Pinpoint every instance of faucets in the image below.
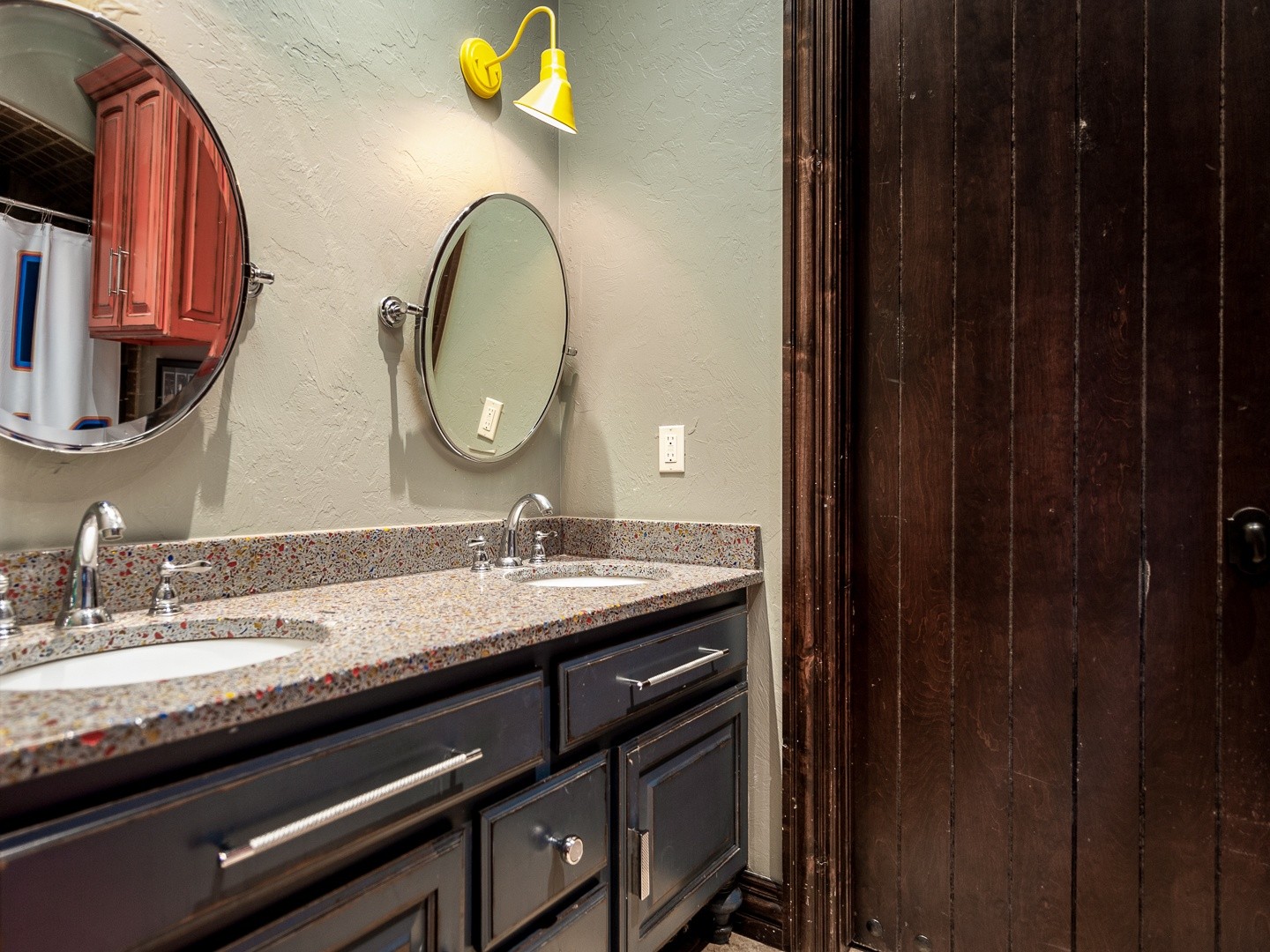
[50,501,126,631]
[489,492,554,569]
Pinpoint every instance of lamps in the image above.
[460,6,577,134]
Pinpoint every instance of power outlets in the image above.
[658,425,686,473]
[477,396,504,442]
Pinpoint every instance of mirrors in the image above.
[0,0,274,457]
[378,192,578,466]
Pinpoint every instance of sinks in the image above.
[0,613,333,693]
[505,559,675,588]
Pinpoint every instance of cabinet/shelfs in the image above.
[71,53,245,346]
[0,585,752,951]
